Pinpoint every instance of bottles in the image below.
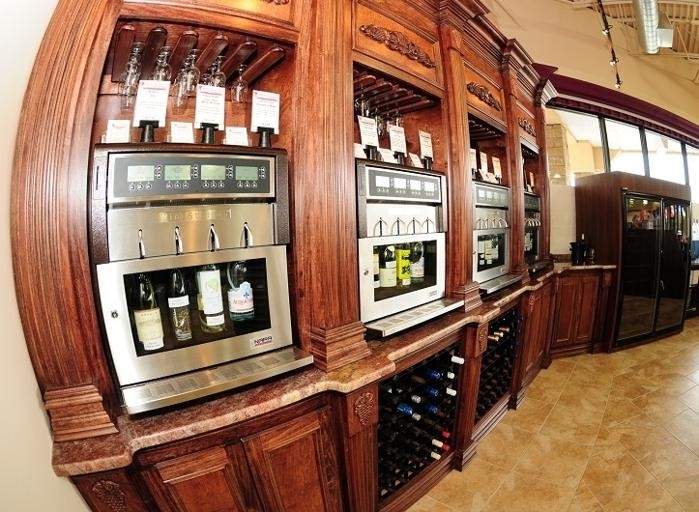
[226,261,257,323]
[127,272,166,352]
[372,241,426,291]
[193,263,227,335]
[396,326,512,464]
[166,267,194,344]
[479,234,500,266]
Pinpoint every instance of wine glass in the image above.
[115,42,250,111]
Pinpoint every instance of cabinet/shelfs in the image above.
[521,271,607,383]
[573,170,693,355]
[122,402,351,510]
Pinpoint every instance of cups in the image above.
[354,84,405,138]
